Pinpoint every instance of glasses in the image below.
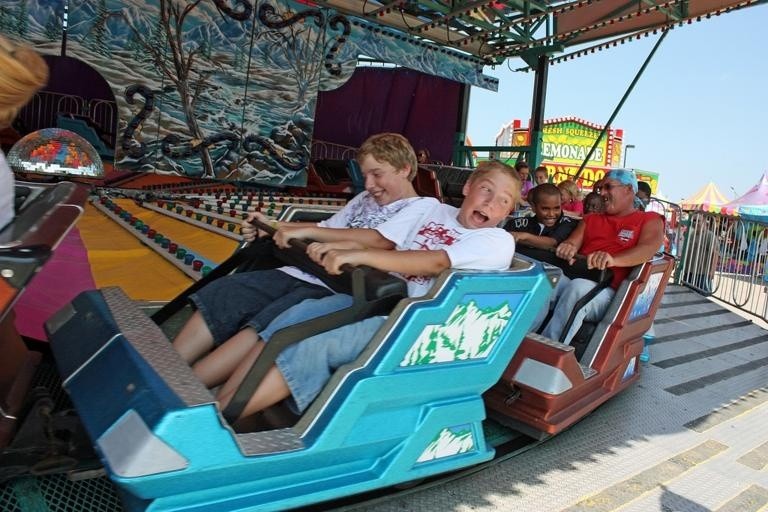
[595,184,627,192]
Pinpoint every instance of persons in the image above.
[213,159,525,420]
[0,35,50,234]
[170,131,437,389]
[497,160,668,343]
[664,211,768,292]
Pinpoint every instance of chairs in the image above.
[220,213,674,450]
